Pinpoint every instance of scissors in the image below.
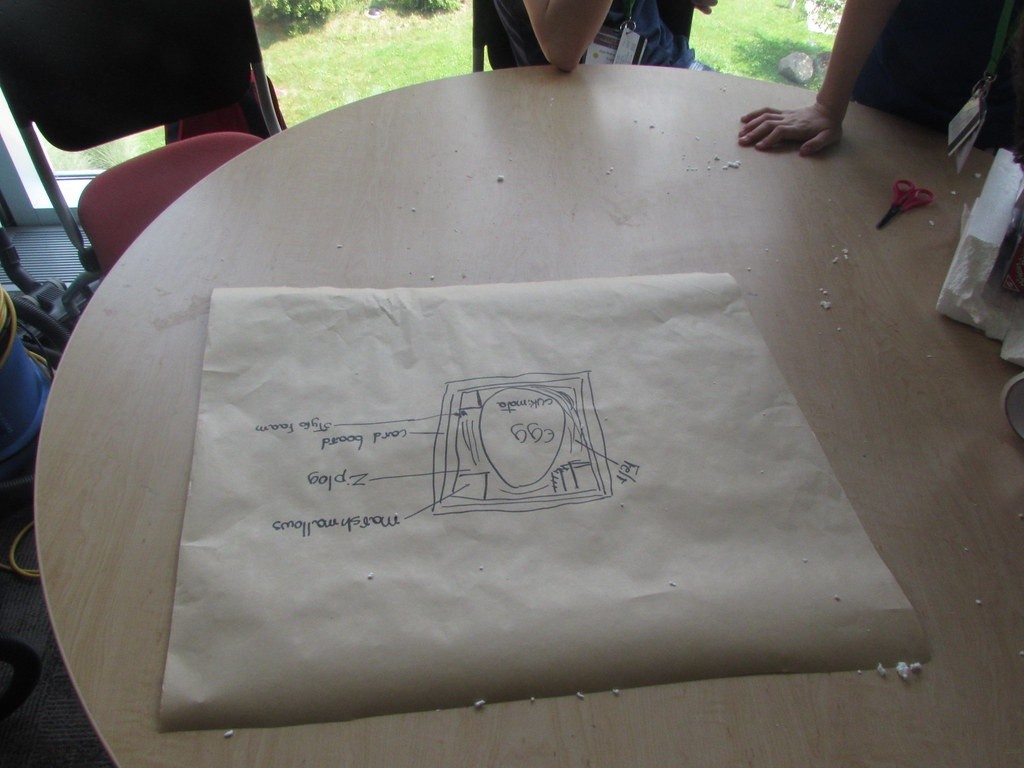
[876,179,936,230]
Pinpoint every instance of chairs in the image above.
[1,0,288,322]
[473,0,694,74]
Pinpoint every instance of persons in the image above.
[737,1,1024,156]
[486,0,720,74]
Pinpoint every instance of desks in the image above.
[32,63,1022,768]
